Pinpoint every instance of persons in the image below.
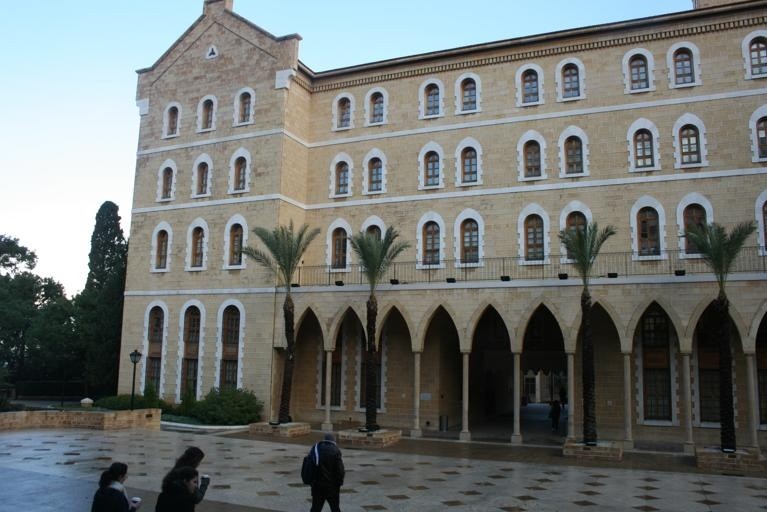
[307,432,344,512]
[559,386,568,410]
[548,400,561,434]
[155,465,198,512]
[162,447,210,504]
[91,462,142,512]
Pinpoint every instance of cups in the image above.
[131,497,141,510]
[201,475,211,486]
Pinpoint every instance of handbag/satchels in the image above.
[301,456,312,485]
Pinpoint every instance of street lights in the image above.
[129,349,142,410]
